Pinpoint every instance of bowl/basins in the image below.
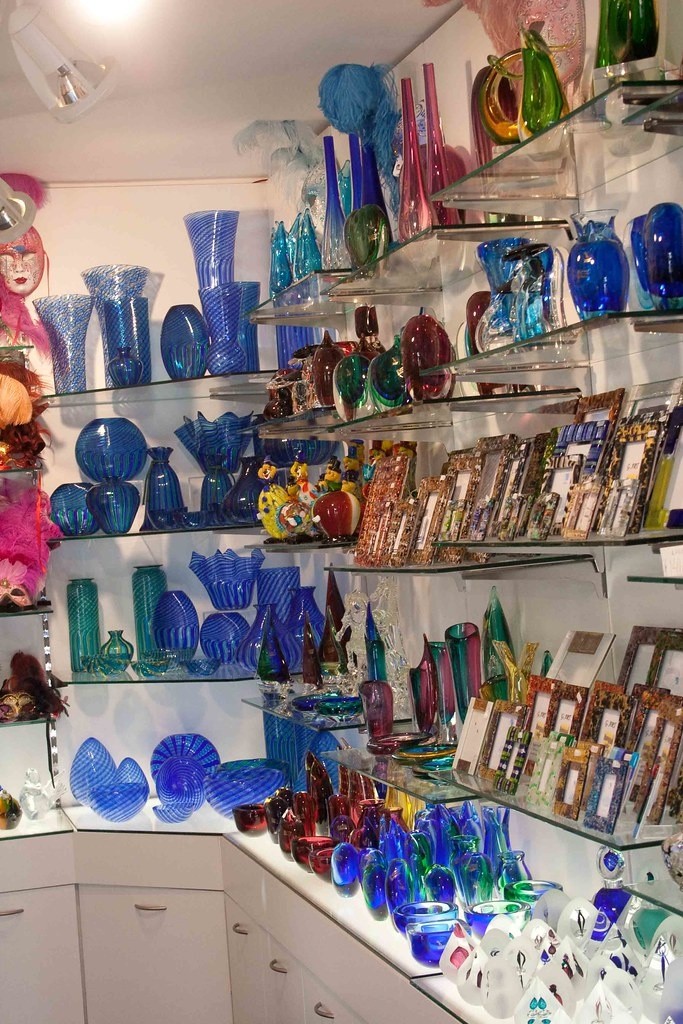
[81,649,223,680]
[308,847,361,884]
[391,901,533,965]
[293,836,333,871]
[233,803,267,837]
[147,504,211,530]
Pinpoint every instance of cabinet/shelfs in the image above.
[34,368,278,833]
[224,891,266,1024]
[240,269,418,734]
[303,961,368,1024]
[77,882,233,1024]
[619,84,683,916]
[309,220,615,806]
[263,928,306,1024]
[0,883,85,1024]
[0,344,74,844]
[429,79,683,853]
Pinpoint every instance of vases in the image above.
[278,745,565,968]
[65,547,362,725]
[33,210,262,395]
[269,62,463,296]
[358,583,553,747]
[48,411,338,537]
[69,690,333,884]
[265,202,683,423]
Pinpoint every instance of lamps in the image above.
[0,177,36,243]
[7,0,121,124]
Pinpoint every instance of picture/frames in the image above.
[451,626,683,836]
[354,376,683,568]
